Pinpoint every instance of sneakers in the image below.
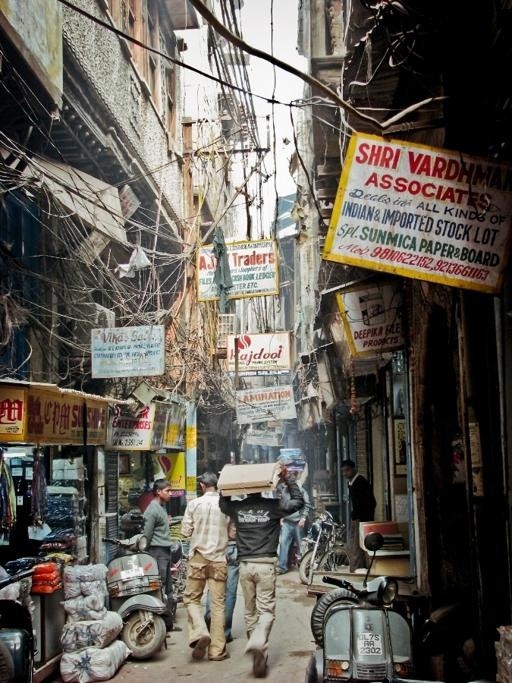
[166,627,268,678]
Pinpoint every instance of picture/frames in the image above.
[393,416,407,477]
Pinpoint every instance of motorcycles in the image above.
[304,532,432,682]
[96,534,188,659]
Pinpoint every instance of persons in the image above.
[339,460,377,573]
[218,460,305,678]
[203,521,238,642]
[180,471,235,661]
[142,478,181,631]
[275,466,310,575]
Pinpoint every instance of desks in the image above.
[362,544,410,576]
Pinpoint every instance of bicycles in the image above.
[298,504,353,585]
